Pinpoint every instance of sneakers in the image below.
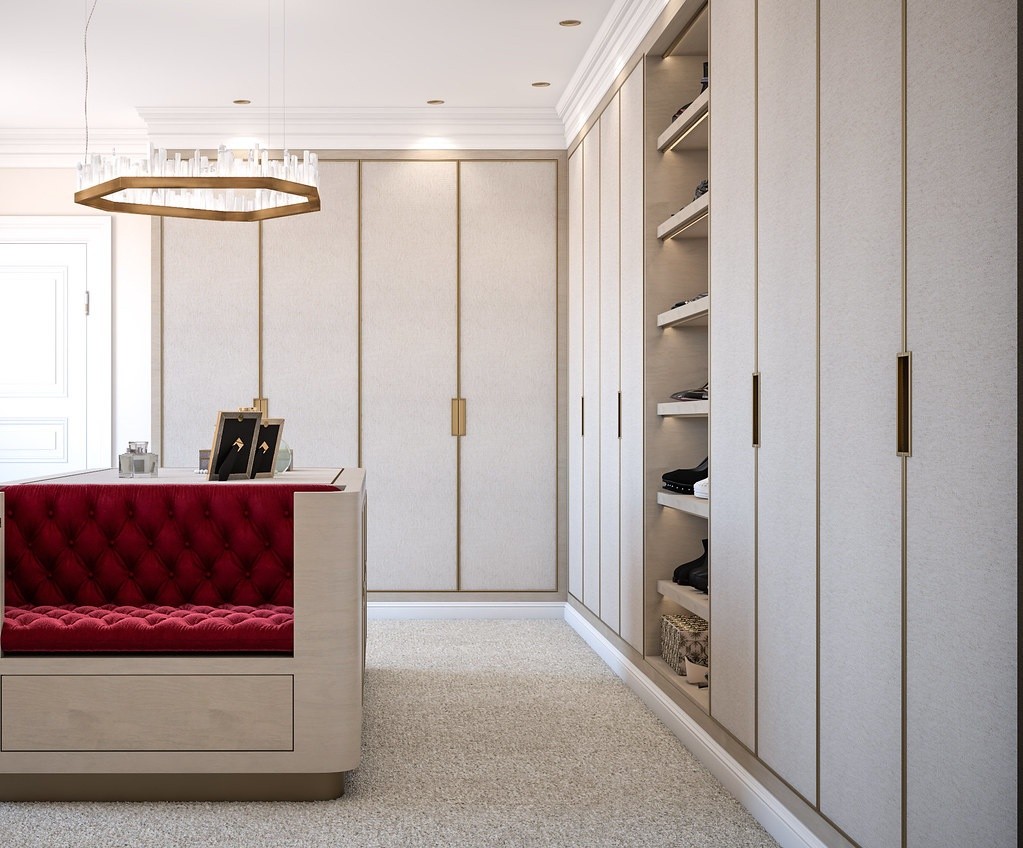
[670,380,709,401]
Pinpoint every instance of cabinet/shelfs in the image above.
[150,1,1023,847]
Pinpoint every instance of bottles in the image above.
[118,441,159,478]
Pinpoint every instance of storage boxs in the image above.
[662,614,709,675]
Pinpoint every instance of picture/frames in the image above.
[208,411,262,481]
[250,418,285,478]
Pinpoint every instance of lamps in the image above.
[74,1,321,222]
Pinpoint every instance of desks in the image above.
[0,467,366,804]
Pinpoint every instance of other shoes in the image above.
[661,456,709,495]
[671,539,708,593]
[693,477,709,500]
